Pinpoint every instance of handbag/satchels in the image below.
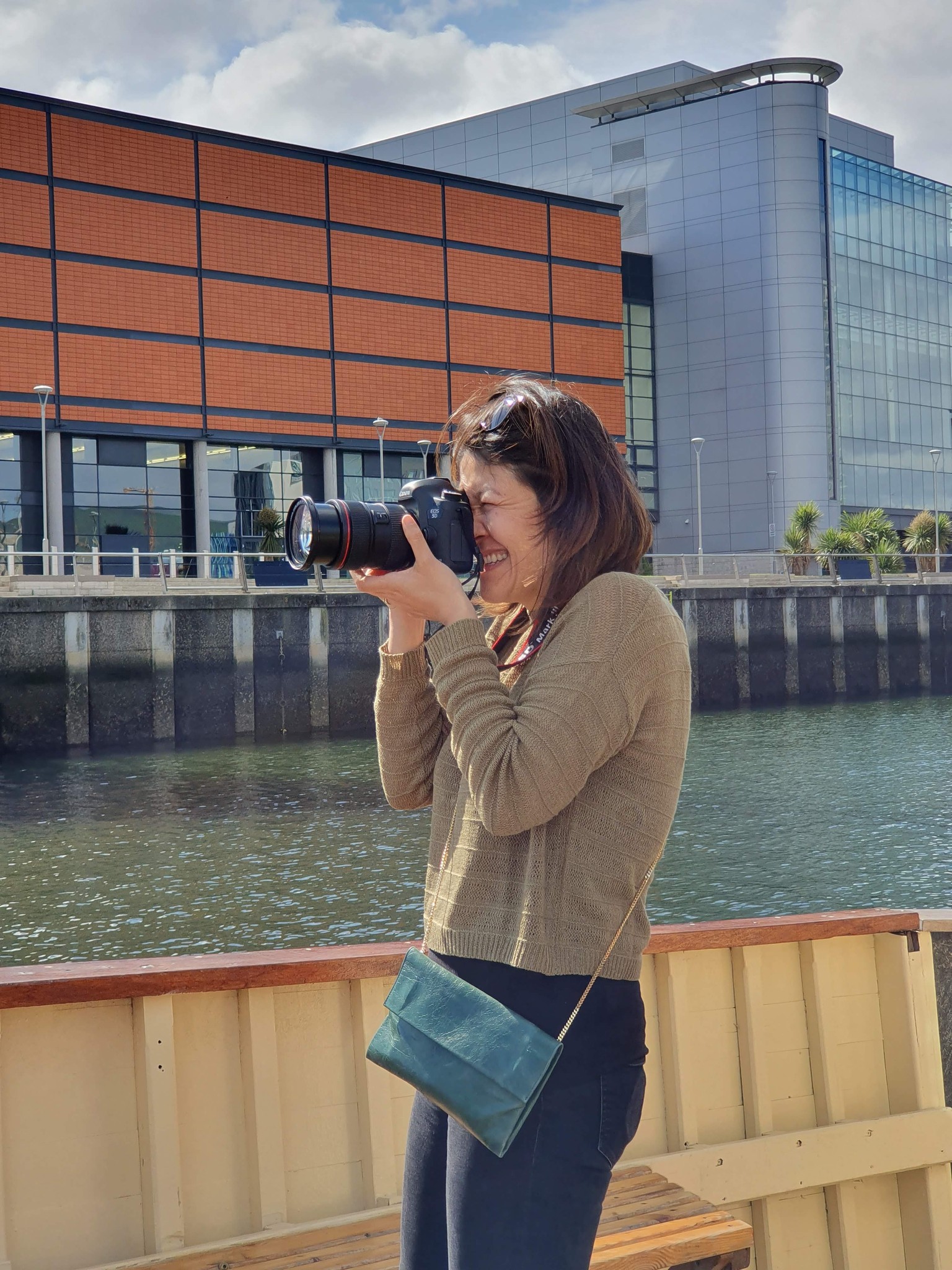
[366,947,563,1158]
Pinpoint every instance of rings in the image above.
[383,598,390,606]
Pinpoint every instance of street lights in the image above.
[418,440,434,479]
[372,416,389,504]
[766,470,778,554]
[34,385,54,576]
[929,448,942,573]
[691,437,705,575]
[0,500,9,552]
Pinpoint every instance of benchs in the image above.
[89,1165,756,1270]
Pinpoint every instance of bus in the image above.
[150,557,184,577]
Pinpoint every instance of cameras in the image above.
[284,476,474,576]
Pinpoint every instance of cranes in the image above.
[124,488,155,552]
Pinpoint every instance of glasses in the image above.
[474,392,532,431]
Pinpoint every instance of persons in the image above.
[347,377,694,1270]
